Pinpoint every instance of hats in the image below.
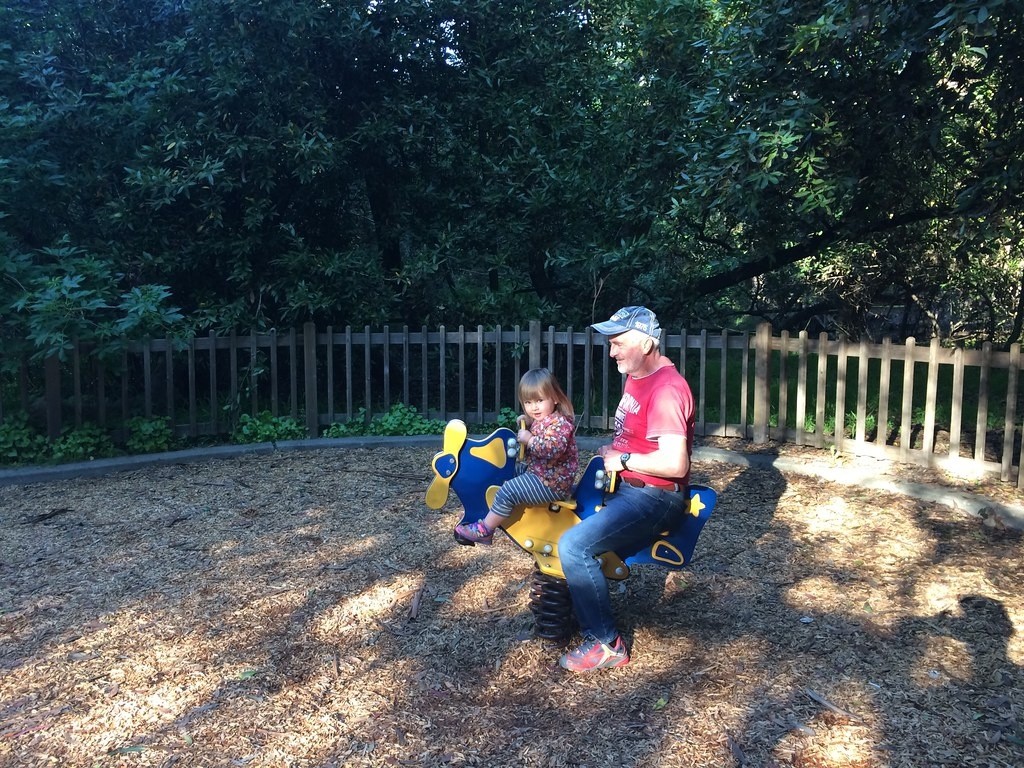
[591,306,662,340]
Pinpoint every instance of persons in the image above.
[455,368,579,545]
[557,306,695,671]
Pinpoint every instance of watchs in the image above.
[620,452,631,471]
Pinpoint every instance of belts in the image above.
[618,475,684,491]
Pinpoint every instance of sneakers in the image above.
[456,519,493,545]
[559,636,628,672]
